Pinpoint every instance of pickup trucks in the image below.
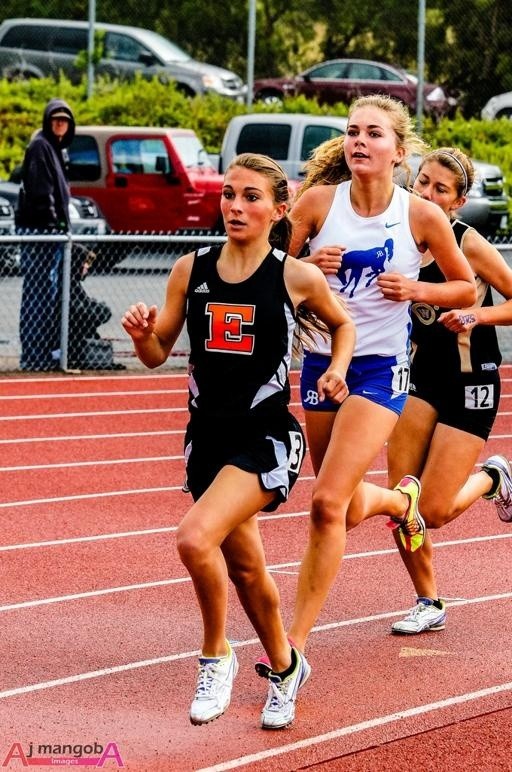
[137,110,505,243]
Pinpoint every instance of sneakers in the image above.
[391,597,446,635]
[254,641,311,728]
[189,640,239,726]
[386,474,425,554]
[482,455,512,524]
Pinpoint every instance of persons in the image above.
[254,92,477,682]
[15,100,75,372]
[122,152,357,728]
[391,149,512,634]
[67,243,112,369]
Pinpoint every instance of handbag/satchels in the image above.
[80,339,114,370]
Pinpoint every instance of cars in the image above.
[481,90,512,125]
[0,182,120,278]
[255,56,461,123]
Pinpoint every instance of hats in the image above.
[51,109,72,121]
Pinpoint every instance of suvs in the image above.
[8,121,304,265]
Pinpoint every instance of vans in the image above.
[0,18,251,104]
